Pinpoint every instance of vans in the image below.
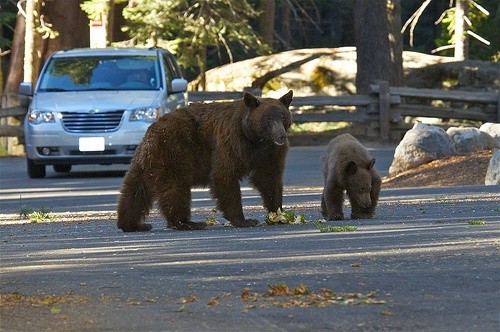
[19,46,190,179]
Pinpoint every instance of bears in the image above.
[320,133,382,221]
[115,90,294,233]
